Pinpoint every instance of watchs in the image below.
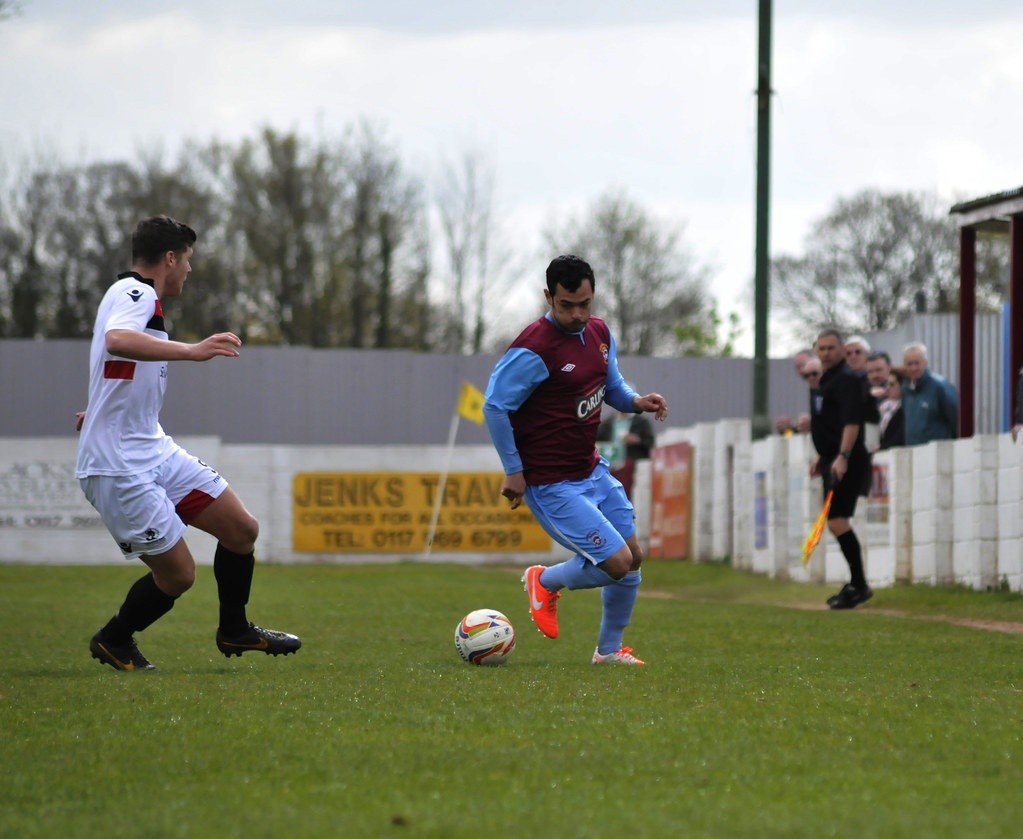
[840,452,850,458]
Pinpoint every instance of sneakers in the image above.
[591,646,646,665]
[90,629,156,672]
[826,583,872,609]
[216,622,302,658]
[521,565,561,639]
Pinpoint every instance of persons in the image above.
[878,369,905,450]
[596,411,654,501]
[482,255,669,665]
[807,329,873,609]
[775,351,823,437]
[900,343,960,447]
[73,214,302,670]
[845,335,892,403]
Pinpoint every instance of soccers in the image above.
[454,608,516,666]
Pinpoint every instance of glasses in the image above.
[846,350,860,356]
[803,371,818,379]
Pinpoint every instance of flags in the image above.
[458,384,486,426]
[803,504,830,566]
